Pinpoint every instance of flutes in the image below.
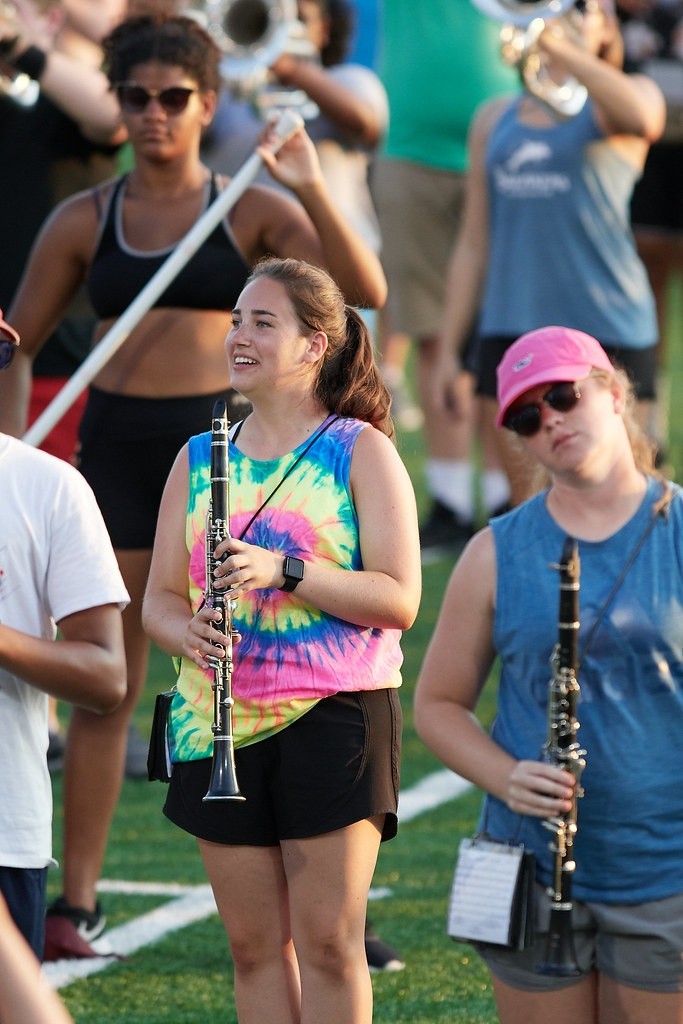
[190,397,247,806]
[527,538,588,981]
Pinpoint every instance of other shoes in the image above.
[414,497,517,561]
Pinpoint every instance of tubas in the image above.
[475,0,604,117]
[203,0,325,127]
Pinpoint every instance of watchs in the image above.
[277,555,304,593]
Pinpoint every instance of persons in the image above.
[411,326,682,1023]
[431,0,666,511]
[0,16,408,971]
[368,0,527,558]
[204,0,388,257]
[0,305,132,974]
[141,258,421,1024]
[0,1,120,758]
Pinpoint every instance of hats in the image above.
[496,327,614,430]
[0,307,20,347]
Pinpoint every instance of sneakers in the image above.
[47,896,106,945]
[364,940,406,973]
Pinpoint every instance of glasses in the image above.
[116,84,203,116]
[0,340,16,371]
[575,0,594,17]
[506,371,607,435]
